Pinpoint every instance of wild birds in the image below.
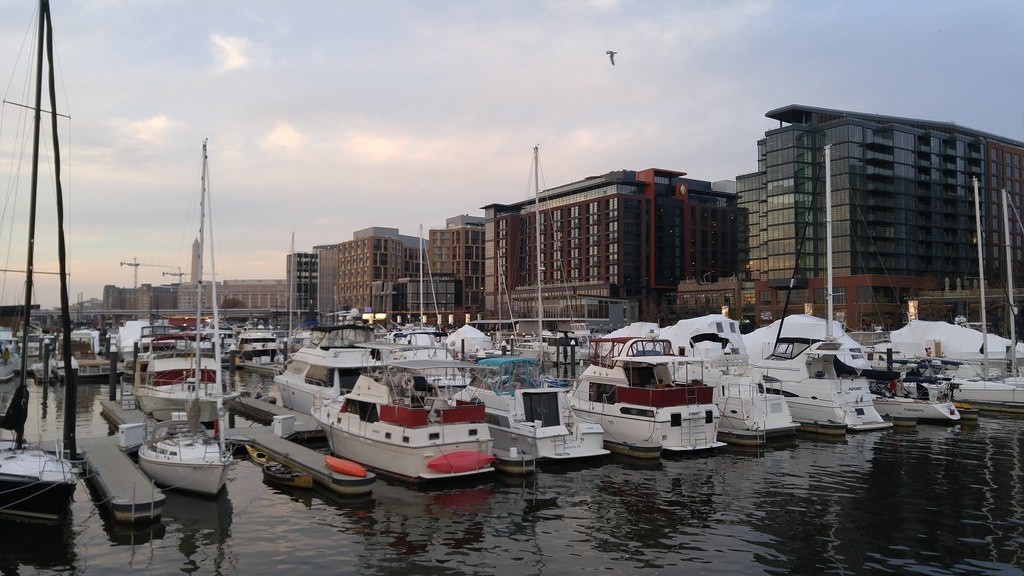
[605,50,618,66]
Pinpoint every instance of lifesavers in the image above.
[400,374,415,388]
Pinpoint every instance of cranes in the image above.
[163,267,219,283]
[120,258,173,286]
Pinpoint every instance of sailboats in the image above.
[138,137,239,494]
[0,0,89,527]
[514,146,601,362]
[383,223,448,343]
[750,144,961,419]
[896,175,1024,402]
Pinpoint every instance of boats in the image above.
[135,333,242,423]
[447,357,611,459]
[383,330,473,387]
[752,342,893,430]
[668,355,801,436]
[13,333,41,373]
[566,356,728,450]
[311,359,498,481]
[0,339,21,381]
[244,445,279,465]
[230,330,278,364]
[273,324,387,415]
[53,331,125,380]
[263,462,313,489]
